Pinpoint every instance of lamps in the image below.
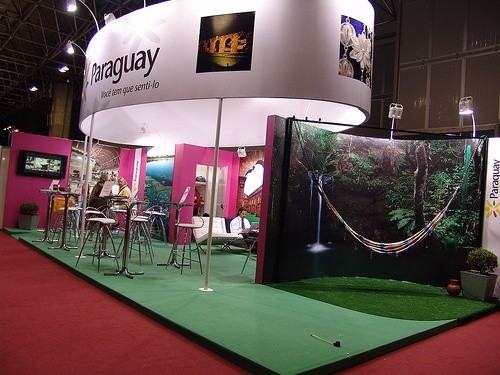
[388,103,404,138]
[58,65,69,73]
[459,97,475,137]
[237,146,247,158]
[67,40,85,58]
[66,0,100,33]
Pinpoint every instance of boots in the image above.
[88,232,94,241]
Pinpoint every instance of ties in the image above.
[241,218,245,232]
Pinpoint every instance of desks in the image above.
[157,202,196,269]
[33,188,80,252]
[101,200,149,279]
[238,230,259,273]
[82,195,128,260]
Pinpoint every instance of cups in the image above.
[129,198,137,218]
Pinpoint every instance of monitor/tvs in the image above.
[16,150,68,179]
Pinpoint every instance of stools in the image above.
[165,216,204,274]
[47,206,168,273]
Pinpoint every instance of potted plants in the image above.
[18,203,40,229]
[460,248,498,300]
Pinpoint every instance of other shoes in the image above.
[109,222,120,231]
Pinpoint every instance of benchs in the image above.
[192,216,249,254]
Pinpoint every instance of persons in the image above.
[194,189,201,215]
[88,174,112,241]
[113,177,131,233]
[230,207,258,253]
